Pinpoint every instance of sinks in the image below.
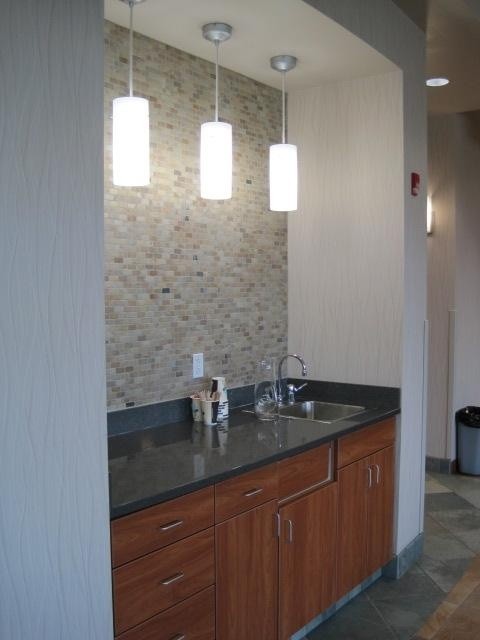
[242,398,379,425]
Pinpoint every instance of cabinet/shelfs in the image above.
[339,418,396,606]
[212,439,340,640]
[112,482,215,639]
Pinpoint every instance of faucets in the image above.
[275,353,306,405]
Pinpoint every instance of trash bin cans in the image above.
[456,406,480,476]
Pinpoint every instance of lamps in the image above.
[108,0,153,190]
[196,21,234,203]
[268,51,301,212]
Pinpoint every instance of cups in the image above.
[255,359,280,424]
[190,377,229,425]
